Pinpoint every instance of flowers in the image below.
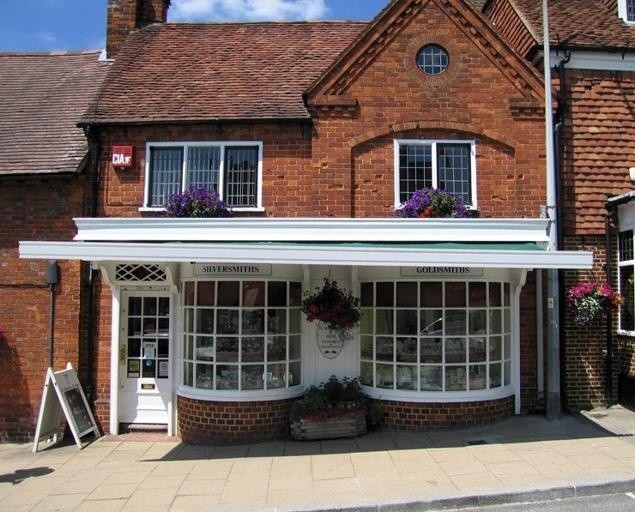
[292,372,371,422]
[160,184,235,218]
[566,280,626,327]
[394,185,470,218]
[300,276,362,333]
